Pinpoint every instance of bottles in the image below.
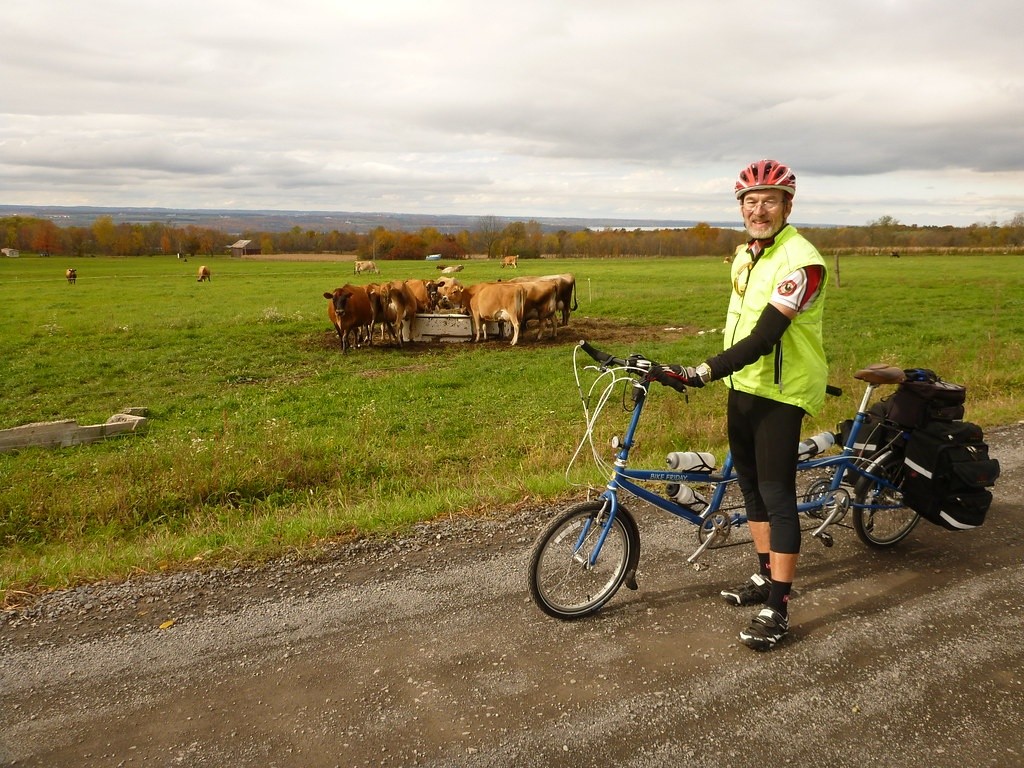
[798,431,836,460]
[666,483,705,511]
[666,452,715,471]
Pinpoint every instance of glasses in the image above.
[741,199,785,211]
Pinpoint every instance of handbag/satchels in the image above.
[840,368,1000,532]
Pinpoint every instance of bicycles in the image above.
[528,340,922,621]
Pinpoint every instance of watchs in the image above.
[696,365,712,383]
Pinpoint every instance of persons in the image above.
[645,160,829,651]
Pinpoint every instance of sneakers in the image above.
[738,605,789,648]
[721,573,772,607]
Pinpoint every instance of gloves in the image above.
[644,364,706,388]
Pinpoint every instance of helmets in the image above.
[735,159,797,200]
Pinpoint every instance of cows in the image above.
[497,274,578,341]
[66,268,77,284]
[436,264,464,273]
[500,255,519,269]
[354,261,380,275]
[323,276,459,355]
[443,282,526,345]
[197,266,211,281]
